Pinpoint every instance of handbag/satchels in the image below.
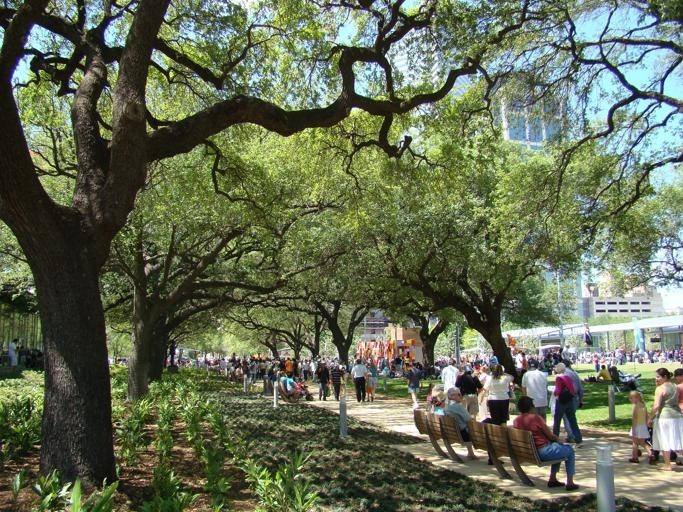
[558,385,575,405]
[508,385,516,399]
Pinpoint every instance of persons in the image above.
[382,347,585,491]
[586,347,683,470]
[8,338,43,369]
[171,347,377,403]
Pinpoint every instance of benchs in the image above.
[468,419,568,486]
[264,379,299,403]
[412,408,472,464]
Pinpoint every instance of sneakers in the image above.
[576,441,584,448]
[412,404,419,410]
[630,458,639,462]
[548,480,565,487]
[650,455,656,465]
[566,484,579,490]
[487,458,504,465]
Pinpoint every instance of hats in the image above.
[527,358,539,368]
